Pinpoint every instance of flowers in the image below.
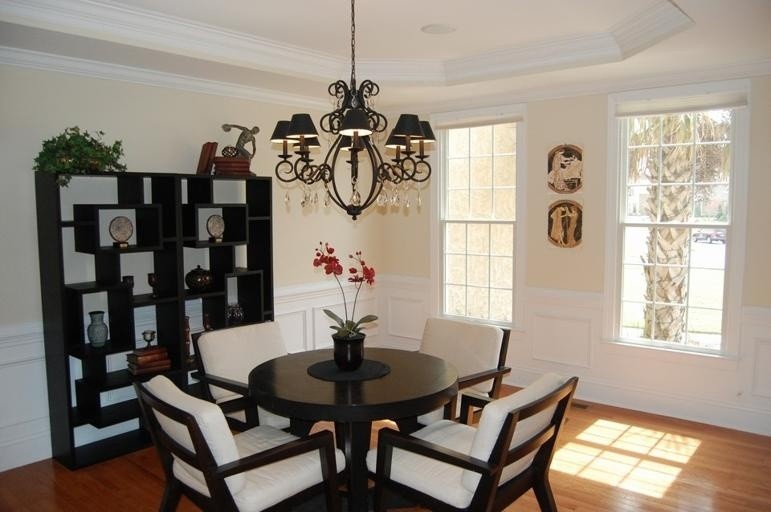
[312,241,378,337]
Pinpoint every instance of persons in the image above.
[551,148,577,190]
[222,123,260,160]
[551,203,578,245]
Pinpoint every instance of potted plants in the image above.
[33,126,127,185]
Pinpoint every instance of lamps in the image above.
[270,0,437,221]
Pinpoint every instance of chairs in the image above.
[363,371,579,512]
[134,374,346,512]
[413,317,511,428]
[191,321,291,433]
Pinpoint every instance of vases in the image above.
[331,332,366,371]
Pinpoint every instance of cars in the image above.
[693,228,727,245]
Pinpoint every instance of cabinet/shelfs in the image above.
[35,171,274,469]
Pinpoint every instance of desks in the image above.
[249,346,458,512]
